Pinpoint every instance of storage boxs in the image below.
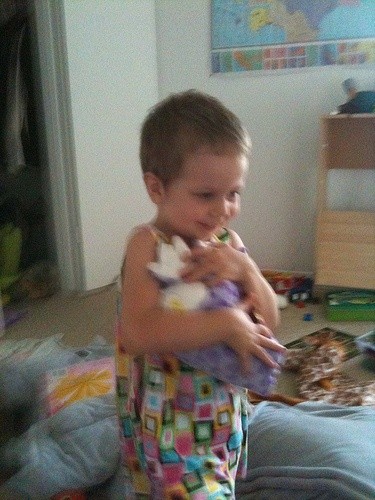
[326,290,375,322]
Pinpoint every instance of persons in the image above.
[114,90,289,500]
